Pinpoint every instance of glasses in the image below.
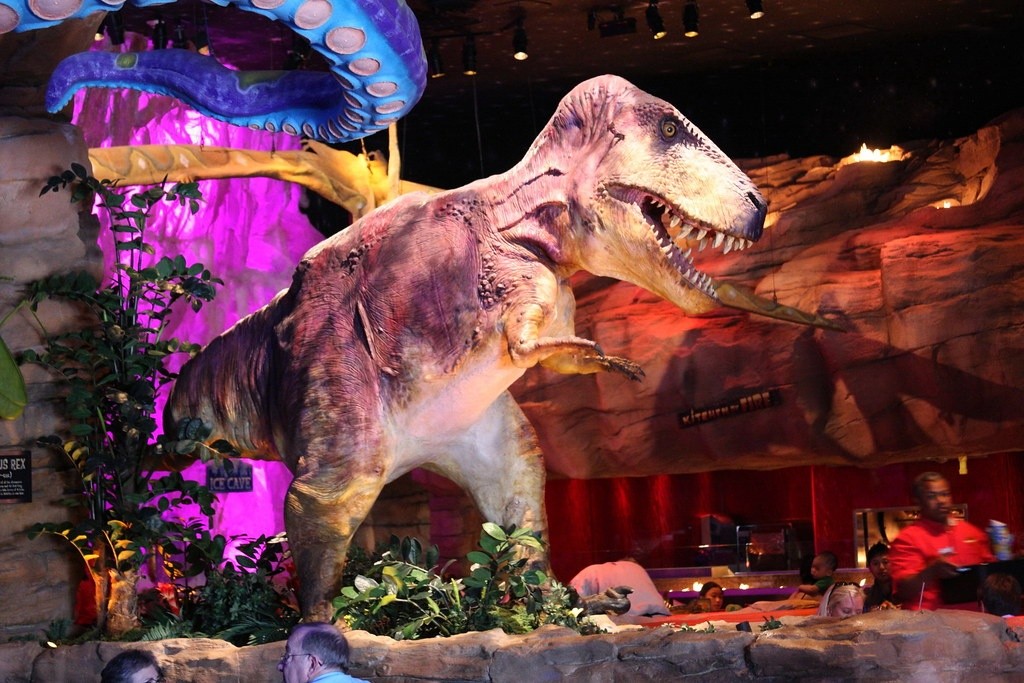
[828,581,861,604]
[279,652,322,668]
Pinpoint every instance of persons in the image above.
[569,558,673,618]
[276,622,370,683]
[797,471,998,618]
[101,649,162,683]
[699,581,724,609]
